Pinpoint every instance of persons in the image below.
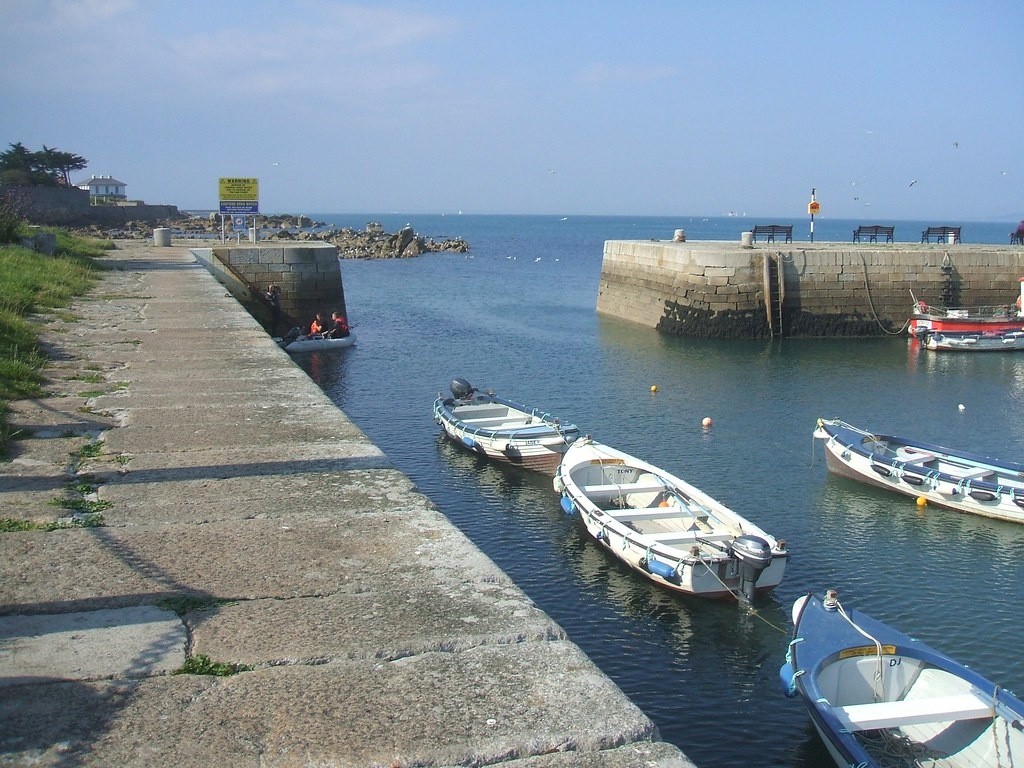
[1015,219,1024,245]
[308,312,328,340]
[322,311,350,340]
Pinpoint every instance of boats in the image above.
[274,326,357,351]
[907,278,1024,338]
[780,590,1023,768]
[812,419,1024,525]
[913,326,1024,350]
[553,433,790,611]
[432,378,581,477]
